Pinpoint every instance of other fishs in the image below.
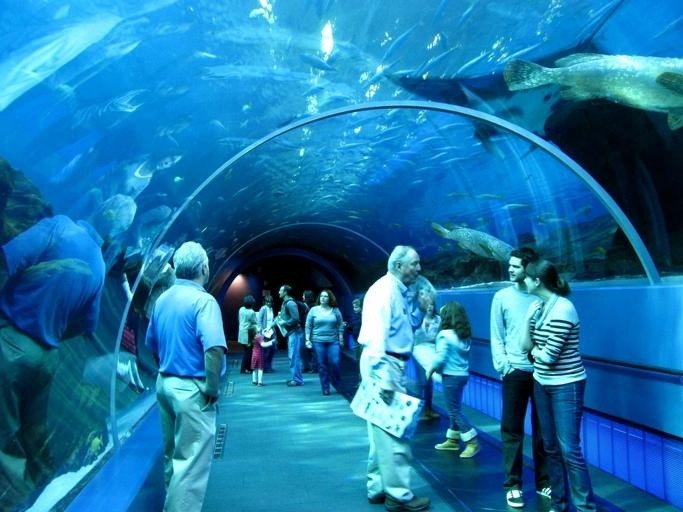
[26,3,558,274]
[24,1,683,274]
[503,50,682,128]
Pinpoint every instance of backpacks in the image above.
[283,299,308,327]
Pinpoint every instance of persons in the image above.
[488,249,554,507]
[145,240,227,512]
[432,298,483,459]
[239,283,441,422]
[359,243,433,509]
[1,0,238,492]
[520,259,598,511]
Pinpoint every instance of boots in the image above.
[435,429,461,450]
[460,428,481,458]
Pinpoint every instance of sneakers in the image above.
[287,379,304,386]
[368,490,430,511]
[324,389,328,394]
[252,382,262,386]
[536,482,552,499]
[507,490,525,507]
[418,408,440,420]
[240,368,253,373]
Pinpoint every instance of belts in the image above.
[386,351,408,360]
